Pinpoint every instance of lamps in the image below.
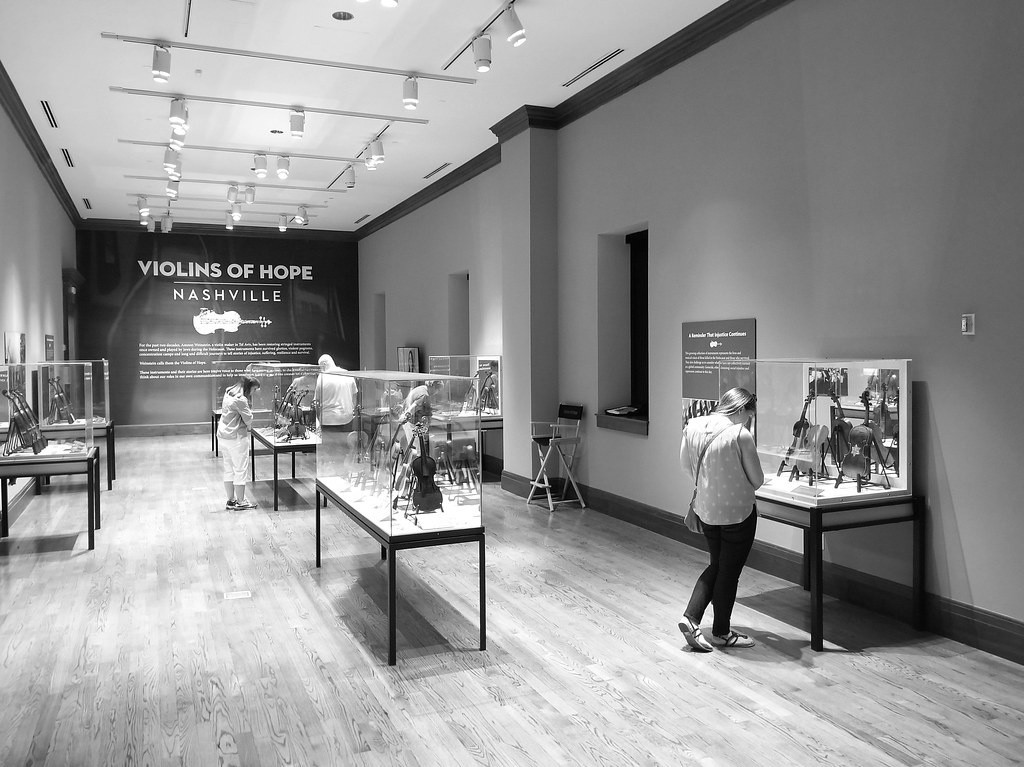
[245,189,254,206]
[163,150,178,167]
[344,169,355,189]
[277,158,290,180]
[169,130,185,147]
[151,50,171,83]
[169,101,186,124]
[370,141,385,164]
[137,199,174,233]
[473,38,492,73]
[290,114,305,138]
[402,80,419,109]
[166,181,179,194]
[501,10,526,47]
[278,216,287,232]
[226,186,242,230]
[295,209,306,225]
[302,212,309,226]
[168,161,182,177]
[254,157,268,179]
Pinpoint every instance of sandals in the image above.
[712,630,755,648]
[678,615,713,652]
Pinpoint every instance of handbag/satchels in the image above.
[684,503,704,535]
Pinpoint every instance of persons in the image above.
[402,380,444,459]
[407,350,416,373]
[679,386,765,651]
[290,354,358,477]
[217,377,261,511]
[483,361,498,408]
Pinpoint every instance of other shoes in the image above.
[226,499,256,511]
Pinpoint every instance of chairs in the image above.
[527,401,586,512]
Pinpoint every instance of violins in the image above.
[48,376,73,419]
[785,389,830,473]
[273,385,309,439]
[828,388,854,463]
[464,371,492,410]
[344,404,443,512]
[840,391,873,479]
[871,383,889,424]
[2,387,41,446]
[863,420,894,466]
[193,307,272,335]
